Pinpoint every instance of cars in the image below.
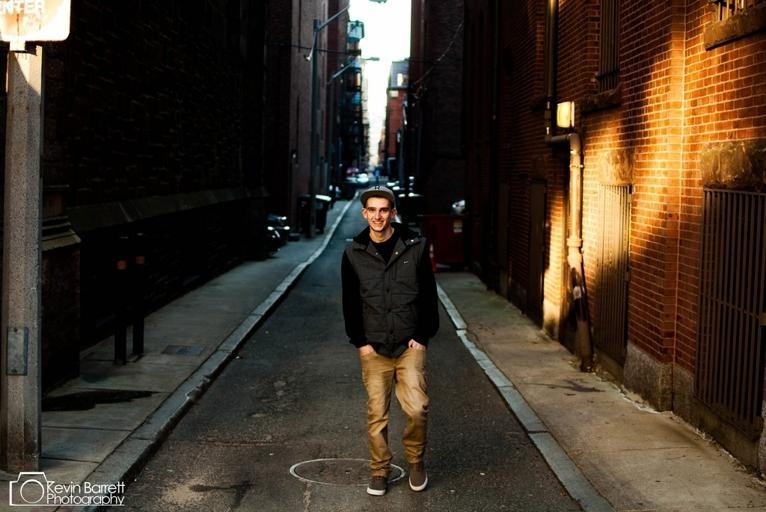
[353,171,370,187]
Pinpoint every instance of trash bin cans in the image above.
[386,181,424,227]
[417,213,465,273]
[297,194,333,234]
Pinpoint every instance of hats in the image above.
[359,185,395,206]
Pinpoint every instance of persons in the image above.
[340,184,438,497]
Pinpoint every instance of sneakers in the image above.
[366,476,387,496]
[408,462,428,492]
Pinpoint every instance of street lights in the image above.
[321,54,382,211]
[304,0,387,238]
